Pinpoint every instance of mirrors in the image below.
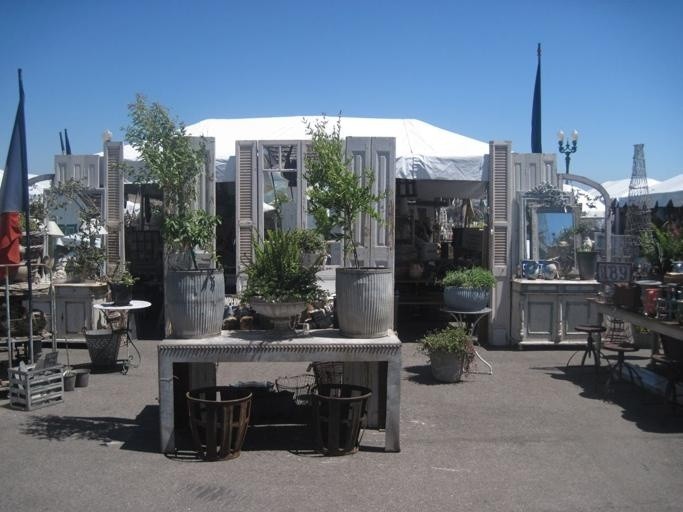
[41,187,106,271]
[529,191,581,279]
[515,187,579,278]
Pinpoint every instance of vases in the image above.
[73,368,90,388]
[62,370,78,391]
[613,261,682,325]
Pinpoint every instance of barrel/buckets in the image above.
[74,367,89,387]
[663,271,682,283]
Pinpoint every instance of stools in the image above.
[640,358,683,417]
[565,324,612,377]
[589,341,643,392]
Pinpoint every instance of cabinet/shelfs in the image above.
[510,276,602,349]
[0,264,57,393]
[25,282,108,346]
[396,280,443,306]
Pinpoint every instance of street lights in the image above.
[554,128,580,186]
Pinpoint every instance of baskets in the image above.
[186,386,254,462]
[276,374,316,407]
[312,362,345,397]
[309,383,372,457]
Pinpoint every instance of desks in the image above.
[92,299,154,374]
[155,330,401,452]
[583,297,682,377]
[439,305,496,378]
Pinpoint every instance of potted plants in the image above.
[105,261,139,305]
[113,91,226,342]
[297,110,395,338]
[238,221,333,340]
[416,326,478,383]
[435,266,495,311]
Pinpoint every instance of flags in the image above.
[0,79,29,282]
[532,54,541,153]
